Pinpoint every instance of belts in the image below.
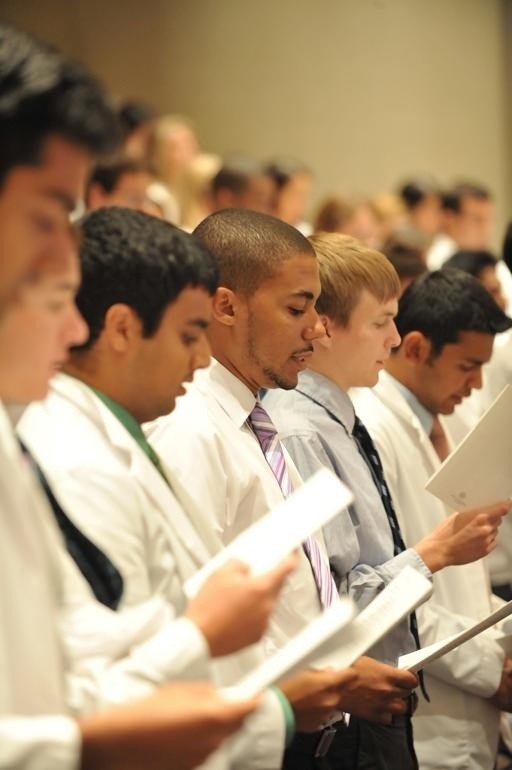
[284,717,350,760]
[391,691,421,724]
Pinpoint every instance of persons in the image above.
[2,30,512,767]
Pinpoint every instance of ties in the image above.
[428,417,452,464]
[14,431,124,612]
[247,402,341,614]
[350,409,431,704]
